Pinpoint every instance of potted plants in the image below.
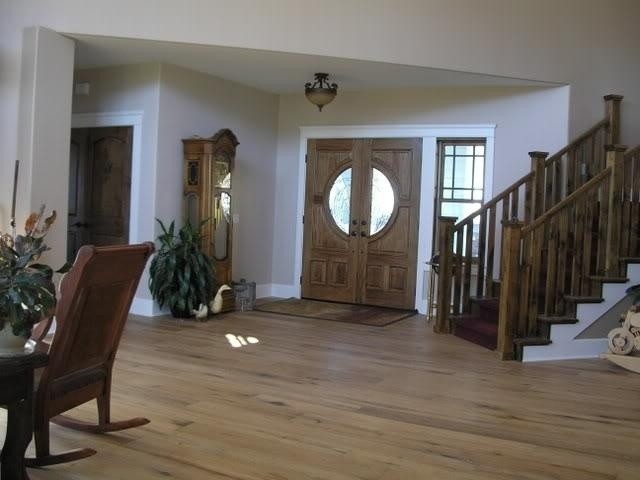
[2,205,58,337]
[149,210,220,318]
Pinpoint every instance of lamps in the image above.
[304,72,338,112]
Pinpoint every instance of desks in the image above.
[0,343,51,479]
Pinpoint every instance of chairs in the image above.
[24,233,154,469]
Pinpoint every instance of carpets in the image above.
[254,298,418,327]
